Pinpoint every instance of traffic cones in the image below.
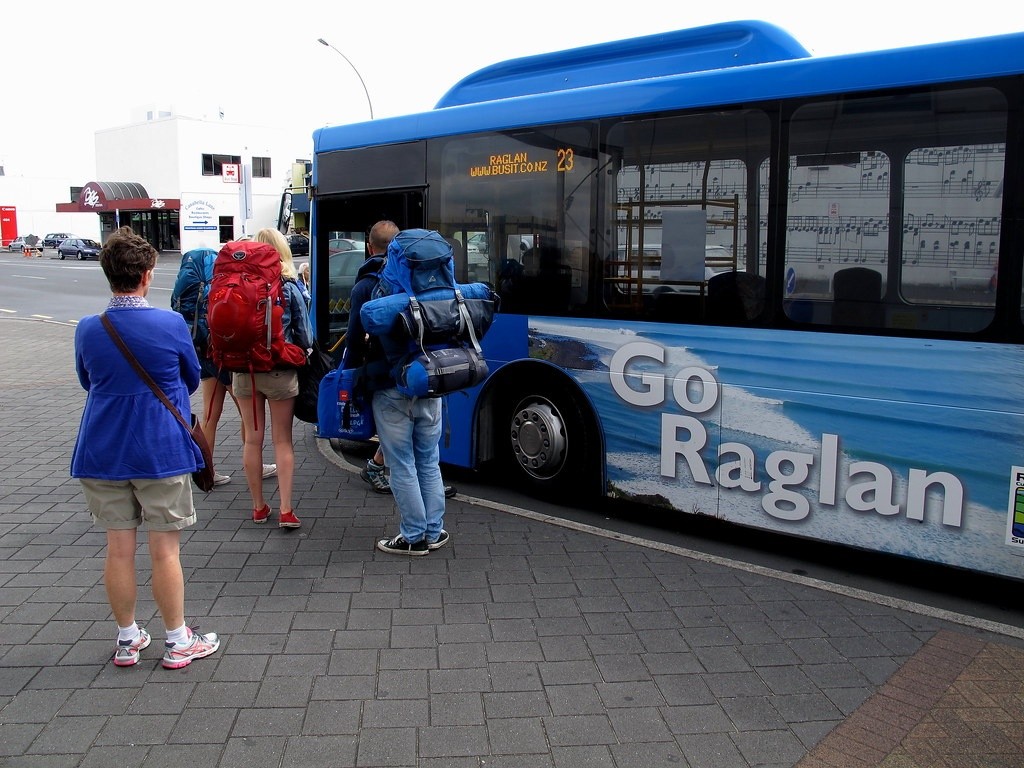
[26,247,33,258]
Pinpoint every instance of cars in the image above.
[615,243,747,296]
[328,250,366,291]
[466,232,539,267]
[286,234,310,258]
[328,237,366,256]
[8,236,44,253]
[43,232,80,248]
[57,238,104,260]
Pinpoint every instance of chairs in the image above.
[833,268,882,326]
[543,265,572,304]
[498,275,542,316]
[707,272,767,327]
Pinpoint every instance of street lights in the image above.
[315,38,374,121]
[24,247,29,257]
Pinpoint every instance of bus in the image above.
[282,19,1023,602]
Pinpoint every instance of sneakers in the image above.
[212,470,231,487]
[424,528,450,550]
[442,486,458,501]
[262,463,278,480]
[377,532,431,556]
[358,458,394,495]
[161,625,222,670]
[112,626,152,666]
[280,507,303,527]
[252,503,272,524]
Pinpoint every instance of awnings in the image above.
[56,181,180,212]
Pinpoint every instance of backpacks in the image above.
[204,239,312,373]
[356,225,505,404]
[168,245,221,351]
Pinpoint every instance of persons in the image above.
[67,225,221,670]
[200,227,310,526]
[346,220,457,557]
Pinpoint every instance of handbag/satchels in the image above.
[312,345,379,443]
[185,411,217,494]
